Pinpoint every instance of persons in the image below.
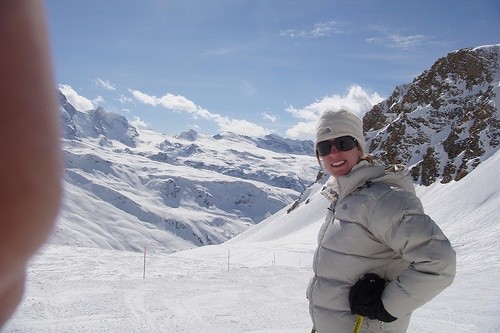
[0,1,65,332]
[306,109,455,333]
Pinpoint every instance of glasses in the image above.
[316,136,357,156]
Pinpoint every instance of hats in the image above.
[314,108,365,157]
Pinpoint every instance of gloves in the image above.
[349,273,397,323]
